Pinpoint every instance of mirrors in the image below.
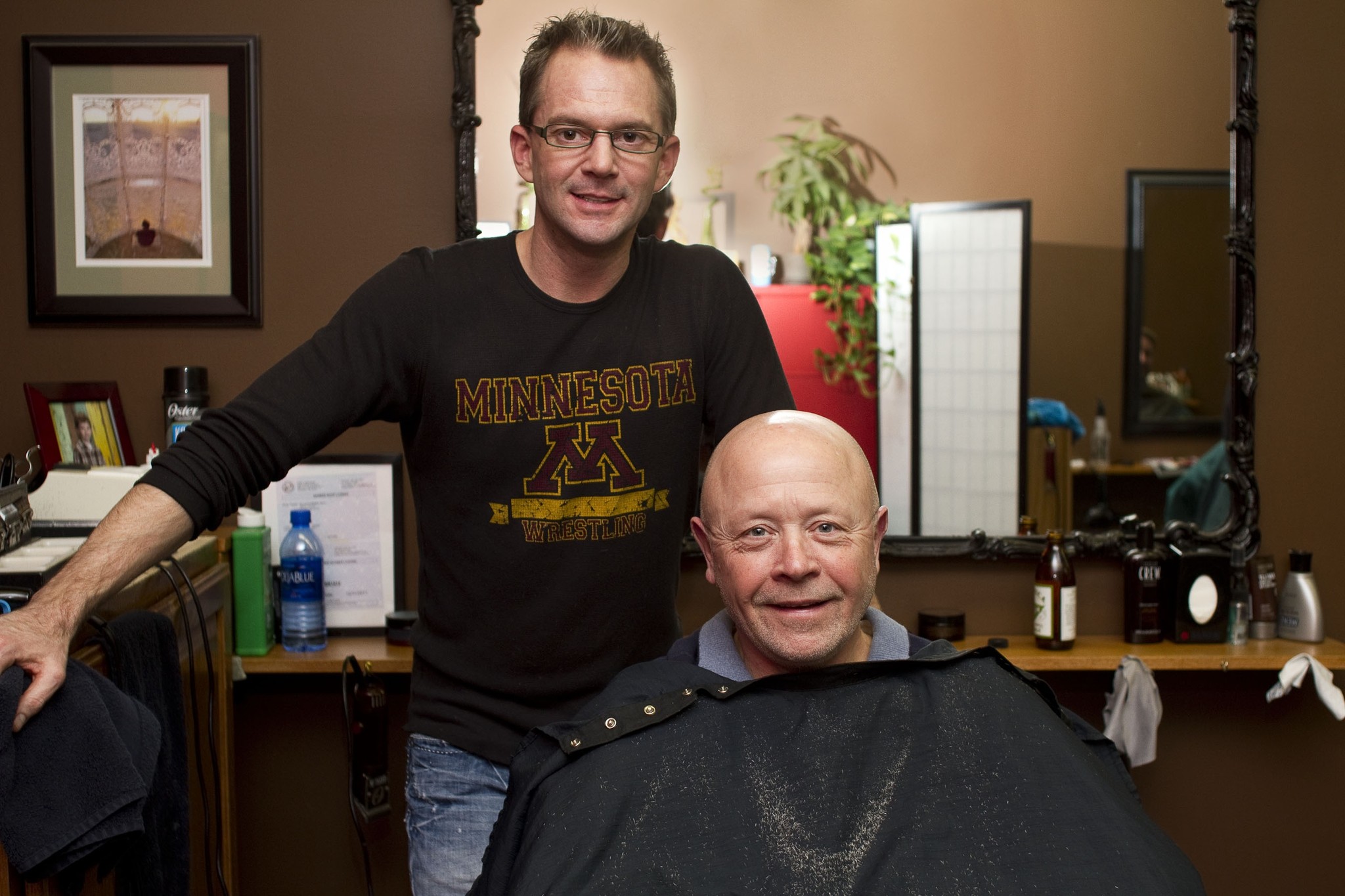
[1113,164,1232,443]
[448,0,1280,569]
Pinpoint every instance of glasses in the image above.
[523,122,665,154]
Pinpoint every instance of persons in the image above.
[0,9,798,896]
[465,404,1214,893]
[71,414,104,466]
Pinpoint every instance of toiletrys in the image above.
[1123,520,1163,646]
[234,507,279,657]
[1089,396,1109,469]
[1279,544,1326,644]
[1229,532,1249,646]
[1249,550,1279,642]
[1031,526,1078,651]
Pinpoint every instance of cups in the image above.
[751,244,778,290]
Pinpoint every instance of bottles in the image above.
[279,509,327,653]
[233,506,277,655]
[1123,522,1166,646]
[161,364,209,453]
[1018,515,1037,536]
[1251,555,1279,639]
[1279,548,1324,641]
[1225,543,1251,647]
[1032,528,1077,652]
[1092,398,1110,468]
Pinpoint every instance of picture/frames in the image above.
[19,33,266,335]
[248,450,406,640]
[24,379,137,470]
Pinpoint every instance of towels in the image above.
[1,656,169,886]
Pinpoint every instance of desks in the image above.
[215,619,1345,729]
